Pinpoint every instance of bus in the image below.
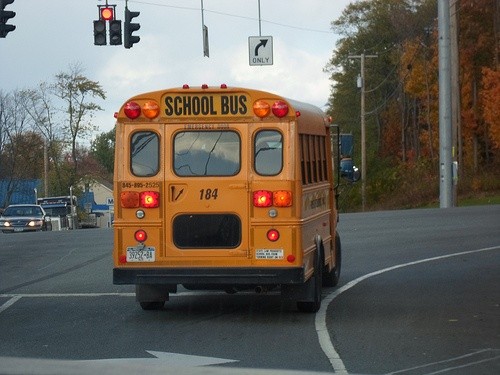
[36,195,77,205]
[111,84,362,314]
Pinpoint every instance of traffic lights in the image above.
[1,0,16,39]
[124,5,141,49]
[93,5,122,46]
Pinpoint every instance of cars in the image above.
[0,204,53,233]
